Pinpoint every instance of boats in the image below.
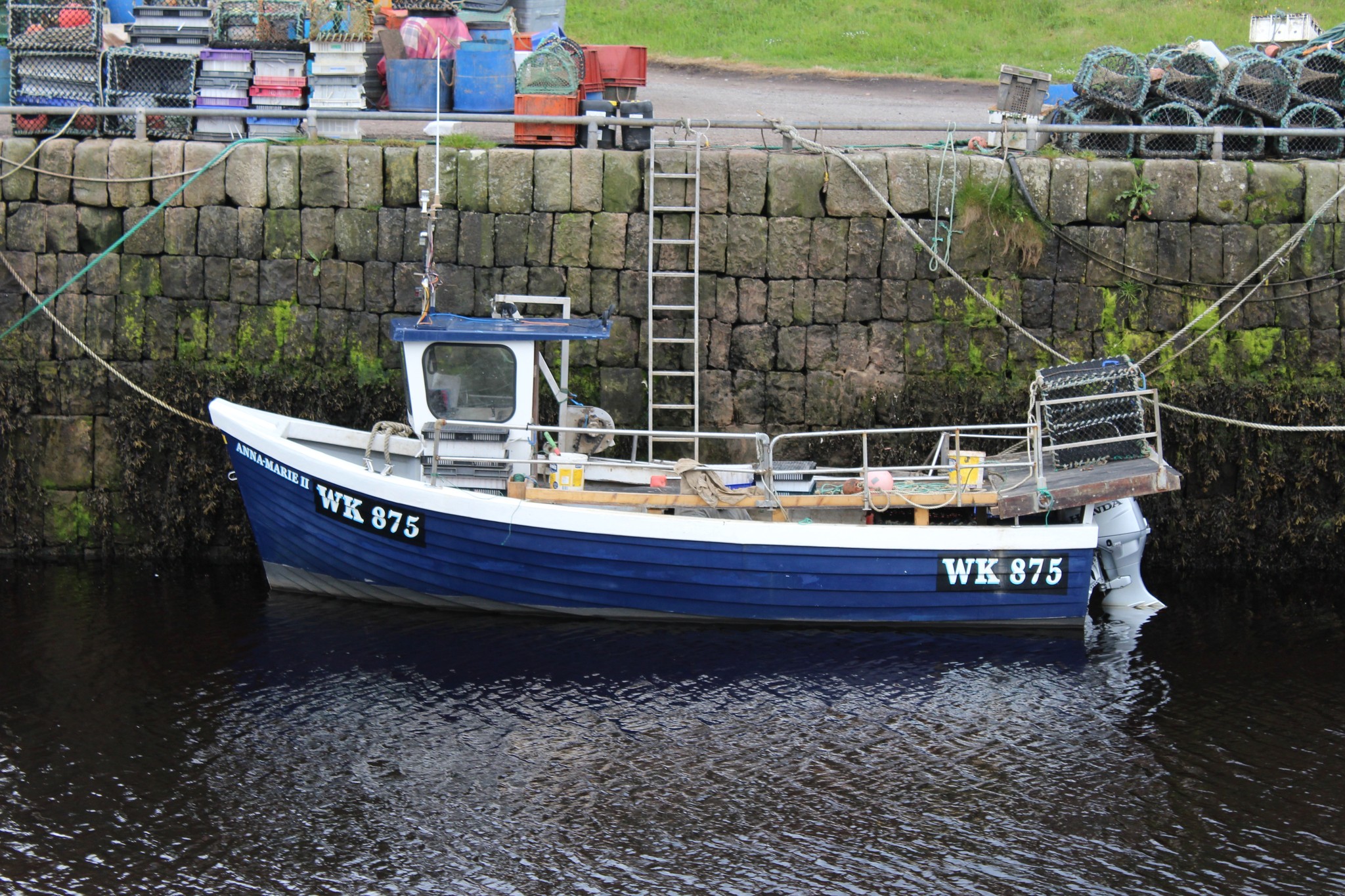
[205,291,1183,631]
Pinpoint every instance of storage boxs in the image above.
[195,41,368,141]
[706,461,817,496]
[997,65,1052,115]
[124,0,211,46]
[1249,13,1324,53]
[16,58,96,108]
[987,103,1059,153]
[510,0,656,149]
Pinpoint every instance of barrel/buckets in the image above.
[453,21,516,114]
[548,452,589,491]
[947,450,987,488]
[384,58,455,114]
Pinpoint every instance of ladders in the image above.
[650,130,701,465]
